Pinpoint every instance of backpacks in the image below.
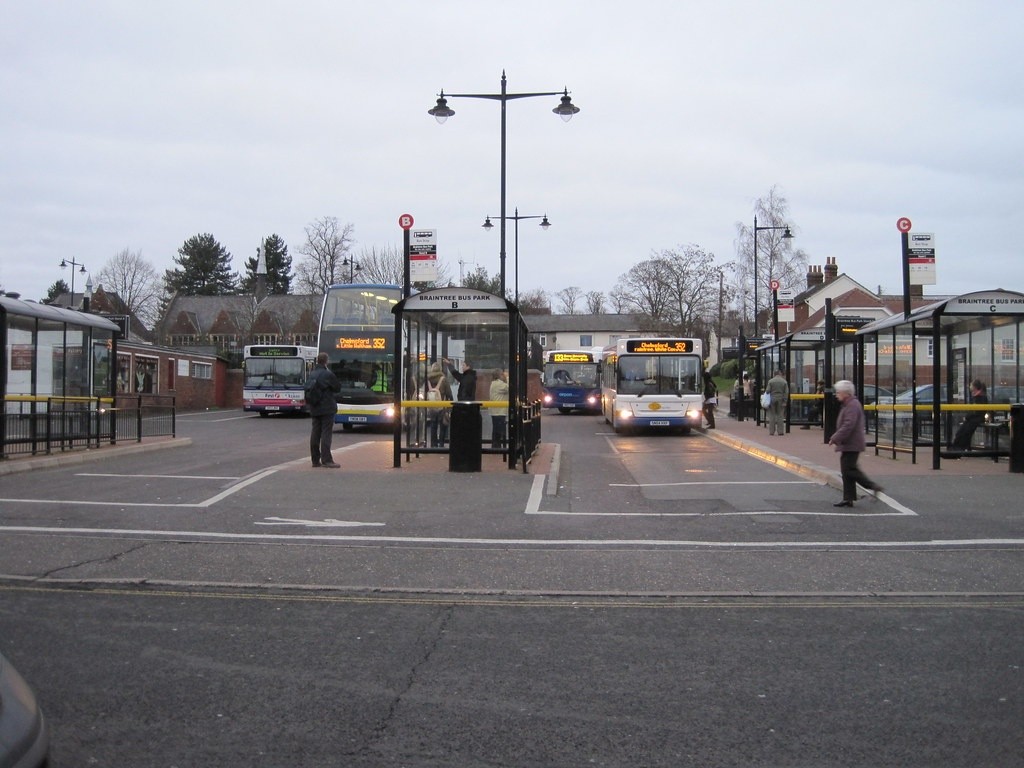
[427,376,445,413]
[302,369,326,407]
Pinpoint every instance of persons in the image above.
[427,362,453,448]
[442,357,478,401]
[556,374,568,387]
[952,379,991,451]
[334,359,366,387]
[304,352,341,468]
[702,372,717,429]
[410,362,426,447]
[490,368,509,448]
[828,380,884,508]
[766,369,789,436]
[734,376,739,400]
[800,380,825,430]
[367,362,387,392]
[743,374,751,400]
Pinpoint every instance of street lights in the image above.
[426,69,580,298]
[754,215,794,336]
[342,255,361,285]
[59,256,87,306]
[481,207,552,307]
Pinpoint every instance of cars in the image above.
[864,384,893,405]
[878,385,948,405]
[988,386,1024,403]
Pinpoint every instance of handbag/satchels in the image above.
[760,391,771,409]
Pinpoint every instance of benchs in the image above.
[975,421,1002,464]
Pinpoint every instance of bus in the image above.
[242,345,317,417]
[541,347,603,414]
[316,283,422,433]
[601,337,708,434]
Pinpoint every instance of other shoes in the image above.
[833,500,853,507]
[706,426,715,429]
[322,461,340,468]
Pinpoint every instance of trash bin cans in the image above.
[450,401,483,473]
[1011,404,1024,472]
